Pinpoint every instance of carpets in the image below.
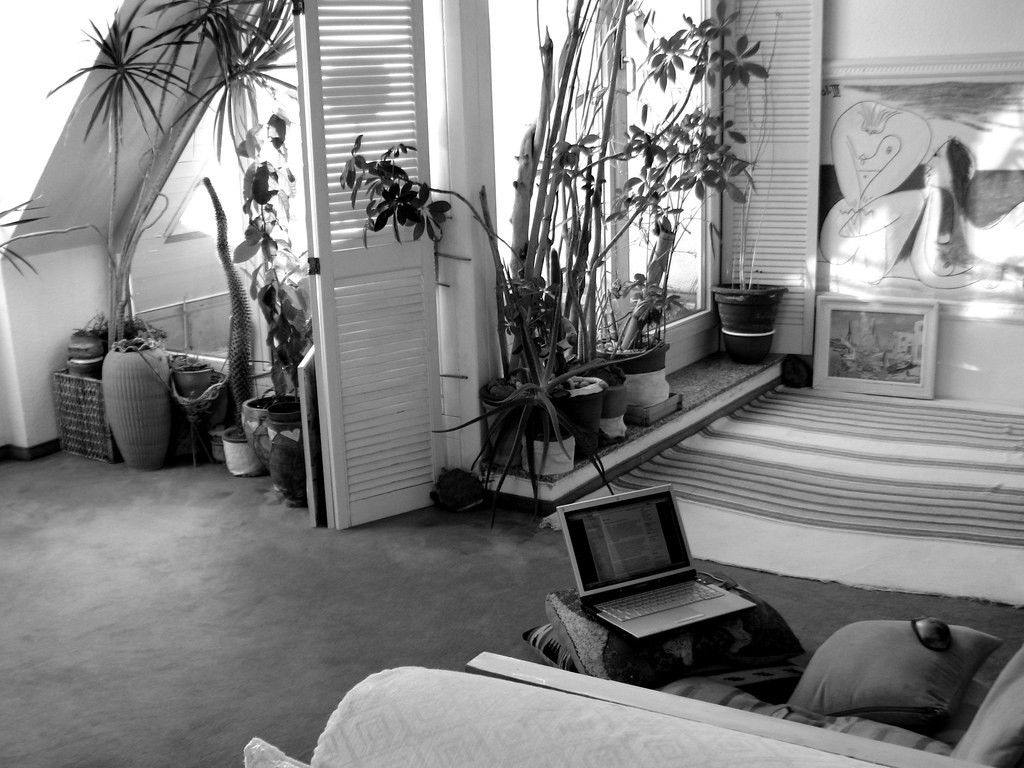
[541,382,1024,608]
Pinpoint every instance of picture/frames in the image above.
[813,294,936,400]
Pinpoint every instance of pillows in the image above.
[788,619,1005,723]
[523,574,805,686]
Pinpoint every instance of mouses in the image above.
[911,616,954,652]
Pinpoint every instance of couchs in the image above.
[239,639,1024,768]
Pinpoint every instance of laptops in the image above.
[557,484,757,641]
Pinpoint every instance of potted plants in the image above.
[0,0,309,510]
[336,0,788,509]
